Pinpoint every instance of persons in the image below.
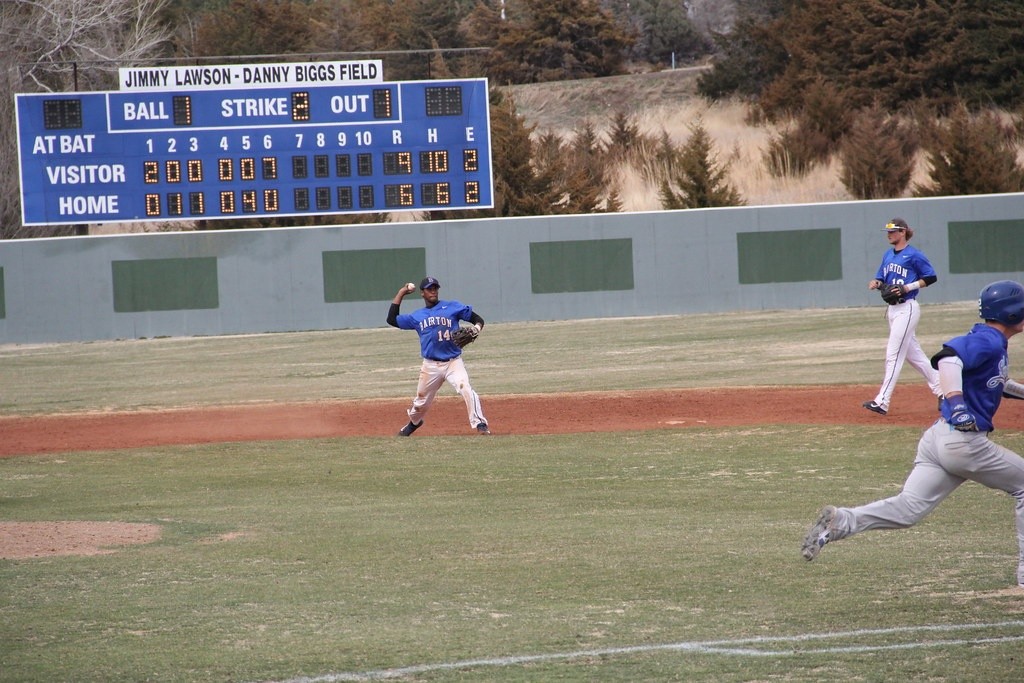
[801,281,1024,590]
[386,276,492,437]
[861,218,945,415]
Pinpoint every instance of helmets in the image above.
[978,280,1024,325]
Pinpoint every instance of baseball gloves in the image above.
[879,283,906,306]
[452,326,479,348]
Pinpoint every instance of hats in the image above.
[420,277,441,289]
[880,217,907,233]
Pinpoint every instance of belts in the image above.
[432,357,456,362]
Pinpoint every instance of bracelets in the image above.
[475,325,481,332]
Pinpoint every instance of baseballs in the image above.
[407,282,416,291]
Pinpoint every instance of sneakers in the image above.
[399,420,423,436]
[937,395,945,411]
[477,423,490,434]
[802,504,850,561]
[862,400,886,415]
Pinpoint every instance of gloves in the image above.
[951,412,980,433]
